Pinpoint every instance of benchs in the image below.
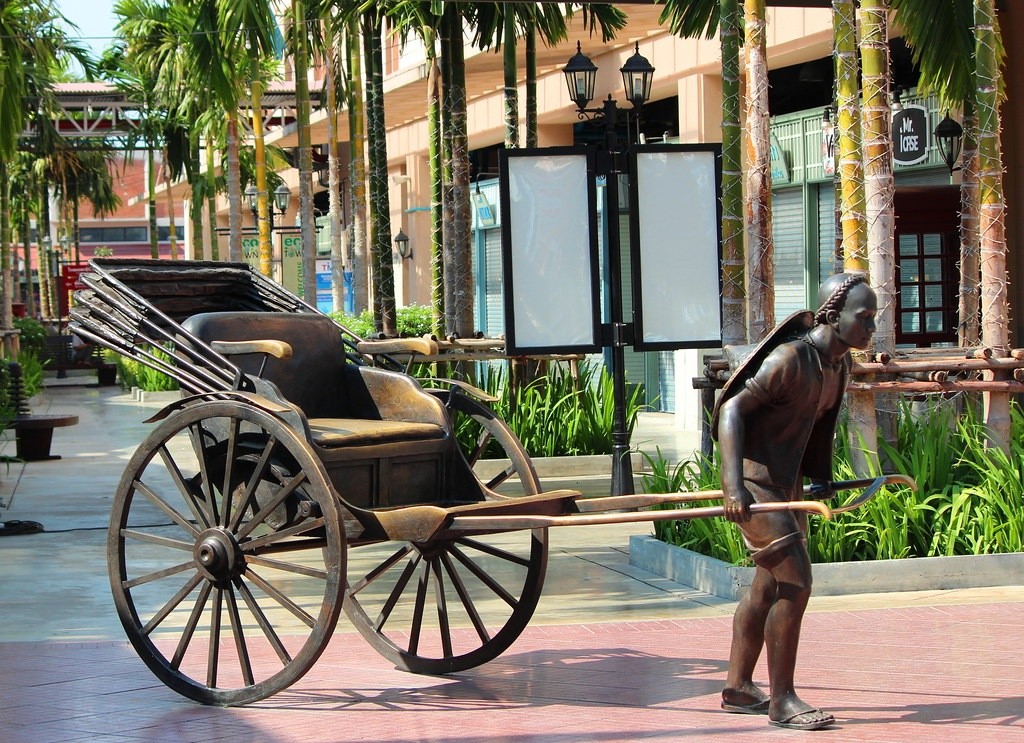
[177,311,452,525]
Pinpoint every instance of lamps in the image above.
[394,227,414,261]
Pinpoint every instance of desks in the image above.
[9,414,78,463]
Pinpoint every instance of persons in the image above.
[718,273,877,730]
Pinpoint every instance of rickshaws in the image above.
[66,256,919,732]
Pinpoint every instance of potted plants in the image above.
[93,342,118,385]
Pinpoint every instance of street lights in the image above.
[245,180,291,262]
[42,234,69,381]
[562,38,655,512]
